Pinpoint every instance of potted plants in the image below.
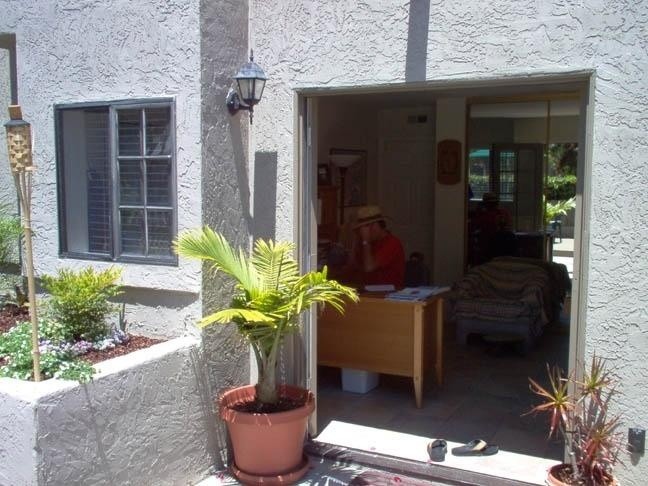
[173,225,360,486]
[523,350,635,486]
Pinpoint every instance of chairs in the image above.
[492,256,572,331]
[405,252,429,285]
[455,261,552,357]
[491,230,519,255]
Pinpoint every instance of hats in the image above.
[351,205,383,229]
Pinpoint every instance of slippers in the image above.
[427,438,447,462]
[452,440,497,456]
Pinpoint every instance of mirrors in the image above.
[465,93,579,335]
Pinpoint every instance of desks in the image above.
[317,287,449,409]
[518,232,552,261]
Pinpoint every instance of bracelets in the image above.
[360,241,370,246]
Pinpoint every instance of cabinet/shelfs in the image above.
[318,185,337,240]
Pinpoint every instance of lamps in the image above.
[330,155,357,223]
[228,49,266,124]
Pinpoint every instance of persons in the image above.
[467,191,515,265]
[336,206,408,290]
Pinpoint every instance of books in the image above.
[364,284,448,304]
[316,239,333,272]
